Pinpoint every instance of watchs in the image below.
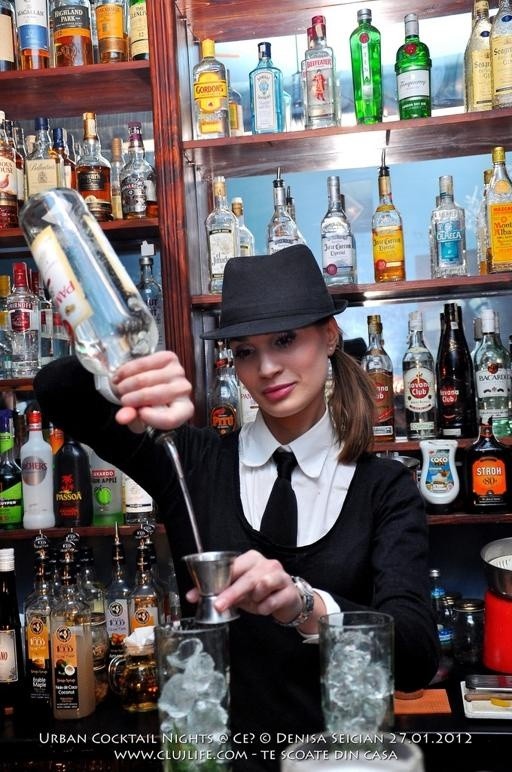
[276,574,316,628]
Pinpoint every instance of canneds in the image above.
[452,599,486,667]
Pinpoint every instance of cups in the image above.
[280,730,424,772]
[154,617,233,771]
[318,610,395,752]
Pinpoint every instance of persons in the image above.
[34,241,442,772]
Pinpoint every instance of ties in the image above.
[257,450,298,549]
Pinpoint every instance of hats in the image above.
[194,245,351,342]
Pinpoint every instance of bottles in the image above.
[207,339,242,438]
[1,0,165,526]
[0,514,181,720]
[429,567,485,669]
[360,302,512,515]
[192,2,512,294]
[17,188,176,445]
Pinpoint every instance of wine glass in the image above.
[184,551,241,627]
